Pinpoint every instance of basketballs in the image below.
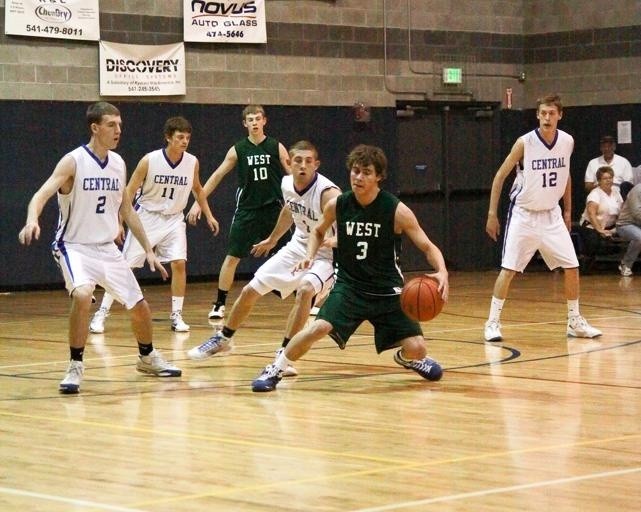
[401,277,444,322]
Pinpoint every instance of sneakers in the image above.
[566,315,603,338]
[275,348,297,376]
[88,310,107,334]
[485,321,503,341]
[59,360,84,393]
[394,349,443,381]
[208,302,225,319]
[618,263,632,276]
[135,348,182,377]
[188,330,232,360]
[171,313,189,332]
[251,363,283,392]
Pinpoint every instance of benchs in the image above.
[573,230,631,268]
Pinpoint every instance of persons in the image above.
[616,183,641,276]
[88,118,219,333]
[186,140,345,377]
[484,93,603,342]
[634,166,641,185]
[585,136,634,201]
[251,144,449,391]
[18,101,182,394]
[578,166,625,271]
[186,104,322,320]
